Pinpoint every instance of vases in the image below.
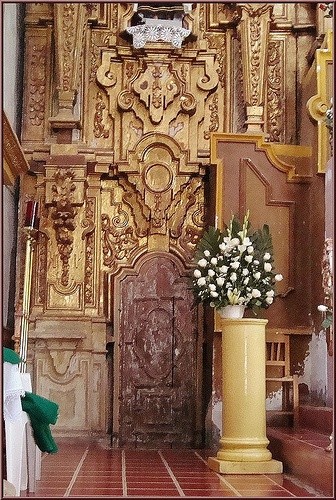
[214,303,248,319]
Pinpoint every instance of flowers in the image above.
[316,303,334,329]
[177,206,284,312]
